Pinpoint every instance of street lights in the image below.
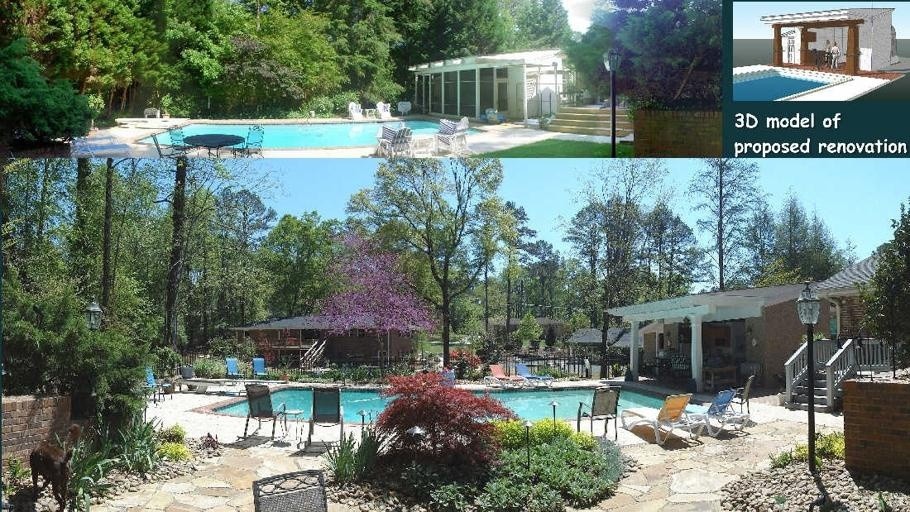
[795,281,821,471]
[83,300,103,420]
[603,46,622,158]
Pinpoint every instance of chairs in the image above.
[150,123,264,160]
[143,367,173,402]
[225,356,267,379]
[252,469,328,512]
[243,383,346,446]
[483,362,553,388]
[375,118,470,158]
[576,374,756,446]
